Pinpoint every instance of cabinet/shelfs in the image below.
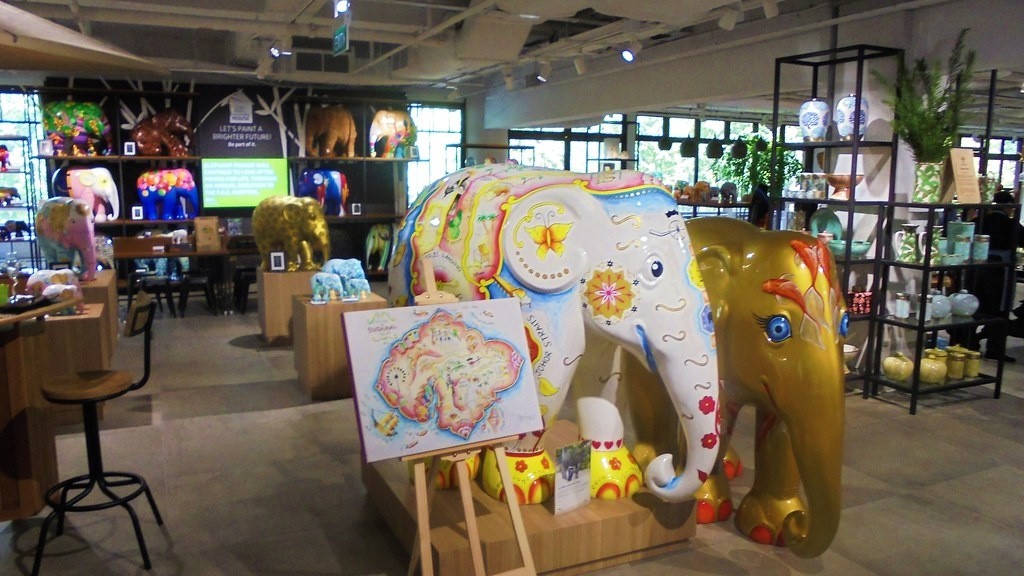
[871,201,1022,417]
[586,121,640,173]
[287,92,420,283]
[0,135,41,271]
[23,85,205,296]
[766,43,906,402]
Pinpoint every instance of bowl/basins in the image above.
[829,240,870,258]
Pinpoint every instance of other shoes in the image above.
[984,353,1016,363]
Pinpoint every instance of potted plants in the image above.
[866,27,985,204]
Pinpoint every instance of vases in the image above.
[835,93,869,141]
[798,96,832,142]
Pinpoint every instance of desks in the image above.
[0,295,84,521]
[112,235,258,318]
[678,197,751,217]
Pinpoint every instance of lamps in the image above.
[537,63,552,83]
[761,0,780,20]
[658,129,768,159]
[573,56,588,75]
[504,76,514,91]
[270,40,282,58]
[281,37,293,55]
[622,41,643,64]
[717,7,740,31]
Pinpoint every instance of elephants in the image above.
[363,224,399,271]
[26,269,83,309]
[300,171,349,217]
[137,168,200,219]
[369,110,417,159]
[43,285,79,314]
[672,181,737,205]
[304,108,357,159]
[0,220,30,239]
[34,197,97,283]
[386,163,723,499]
[252,195,330,270]
[619,215,850,559]
[0,186,21,205]
[810,210,844,241]
[52,165,120,222]
[132,113,195,158]
[41,101,114,157]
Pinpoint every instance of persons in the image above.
[961,190,1024,364]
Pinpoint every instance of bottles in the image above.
[14,272,26,296]
[0,267,14,297]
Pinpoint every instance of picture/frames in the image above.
[351,202,362,216]
[269,250,287,273]
[124,141,136,156]
[132,206,143,220]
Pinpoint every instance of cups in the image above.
[0,284,9,307]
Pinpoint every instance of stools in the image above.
[178,272,217,318]
[29,290,165,576]
[231,266,258,315]
[126,271,164,314]
[139,275,176,320]
